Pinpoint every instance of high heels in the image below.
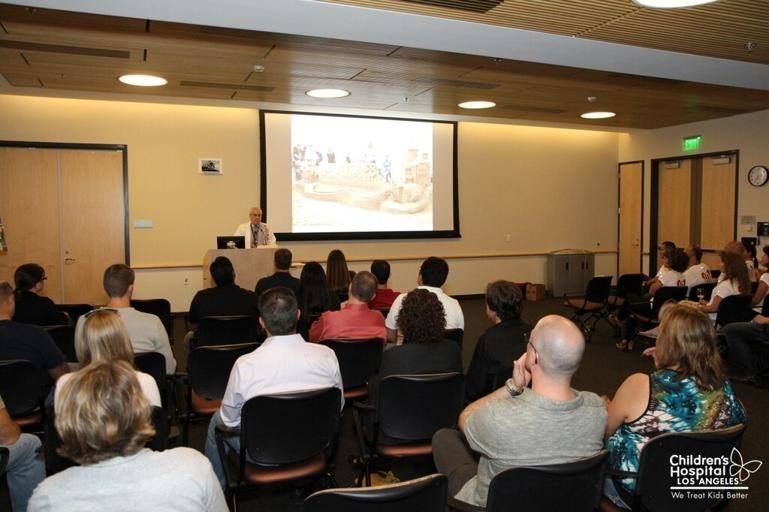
[608,313,626,328]
[616,337,635,350]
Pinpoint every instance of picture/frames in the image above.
[200,158,222,175]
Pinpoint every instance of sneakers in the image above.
[640,327,659,340]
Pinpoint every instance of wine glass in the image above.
[696,289,704,304]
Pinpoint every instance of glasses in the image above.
[523,331,539,366]
[38,277,48,281]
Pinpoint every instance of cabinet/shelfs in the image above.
[547,248,596,298]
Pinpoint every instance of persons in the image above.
[233,207,277,249]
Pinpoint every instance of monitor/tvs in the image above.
[217,236,245,249]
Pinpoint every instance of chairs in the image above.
[0,269,768,512]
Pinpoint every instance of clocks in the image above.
[747,165,769,188]
